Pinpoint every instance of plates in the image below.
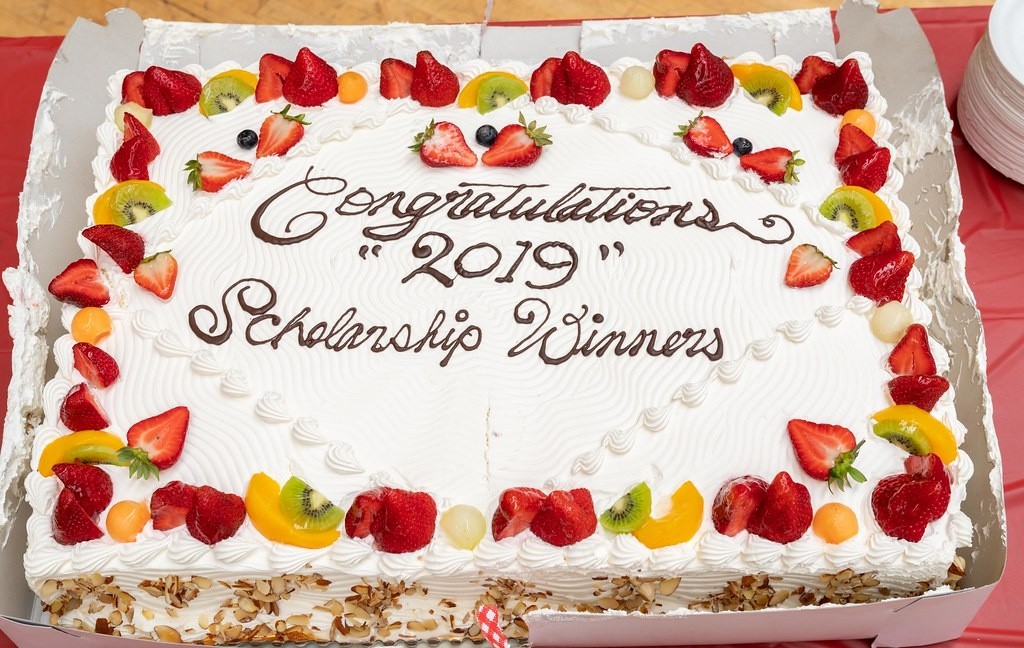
[956,0,1024,186]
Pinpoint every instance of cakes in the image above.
[23,44,973,648]
